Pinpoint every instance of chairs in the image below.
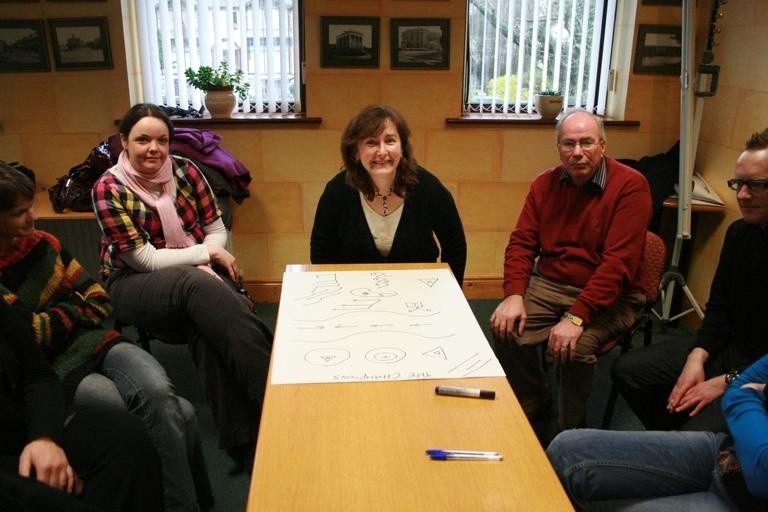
[592,228,665,430]
[114,277,258,357]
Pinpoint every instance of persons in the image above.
[488,106,652,440]
[544,354,767,512]
[308,104,466,289]
[0,293,152,512]
[0,161,198,512]
[90,103,273,475]
[612,129,767,446]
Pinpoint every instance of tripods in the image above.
[644,73,706,323]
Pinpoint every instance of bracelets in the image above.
[563,312,583,327]
[724,370,738,385]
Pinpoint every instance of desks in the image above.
[246,262,575,512]
[662,197,725,325]
[32,190,121,219]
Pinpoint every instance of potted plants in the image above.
[185,61,250,117]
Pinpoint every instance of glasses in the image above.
[727,178,768,192]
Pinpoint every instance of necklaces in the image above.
[375,187,391,215]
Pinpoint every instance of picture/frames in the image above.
[48,15,114,70]
[630,22,682,76]
[0,16,51,73]
[388,16,450,69]
[534,88,564,119]
[318,13,379,68]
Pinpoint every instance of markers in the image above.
[435,386,494,399]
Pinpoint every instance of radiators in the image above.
[35,219,235,284]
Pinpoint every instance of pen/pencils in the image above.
[425,450,502,462]
